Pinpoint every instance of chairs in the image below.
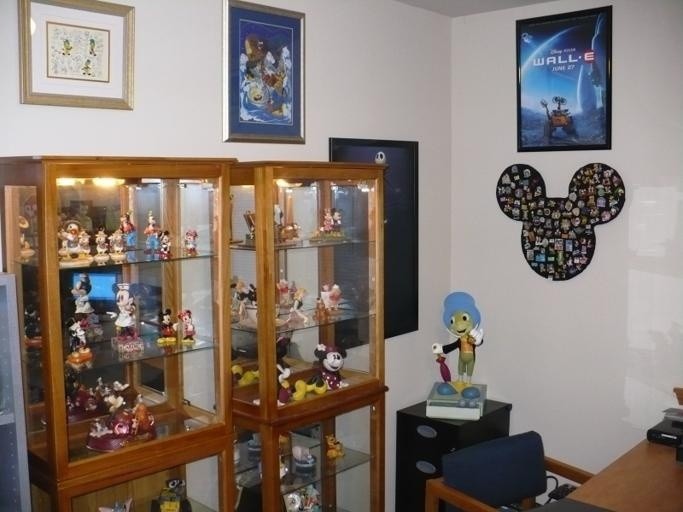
[424,429,597,512]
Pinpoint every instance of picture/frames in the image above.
[221,0,306,145]
[16,0,136,111]
[514,5,613,153]
[325,137,419,350]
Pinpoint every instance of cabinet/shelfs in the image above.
[0,271,33,512]
[209,161,389,512]
[0,154,239,512]
[393,400,512,511]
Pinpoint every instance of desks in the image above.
[526,438,682,512]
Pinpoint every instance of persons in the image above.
[232,278,346,326]
